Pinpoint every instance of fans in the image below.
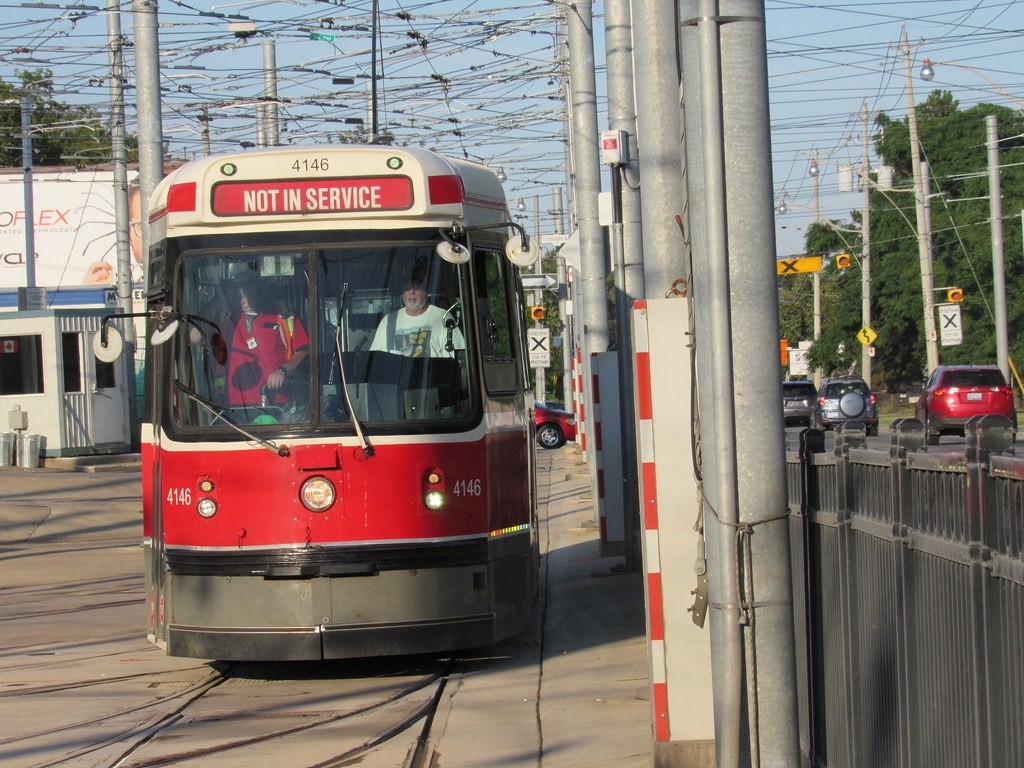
[283,395,308,424]
[320,394,350,424]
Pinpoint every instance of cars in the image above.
[915,365,1018,445]
[782,380,818,424]
[533,400,579,449]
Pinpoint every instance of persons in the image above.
[370,278,465,359]
[190,260,309,421]
[83,171,167,286]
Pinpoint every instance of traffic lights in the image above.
[531,307,545,320]
[835,254,851,269]
[948,288,963,304]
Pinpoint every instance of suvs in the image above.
[809,375,879,436]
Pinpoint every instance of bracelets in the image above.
[278,367,287,378]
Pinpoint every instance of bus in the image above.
[90,145,542,674]
